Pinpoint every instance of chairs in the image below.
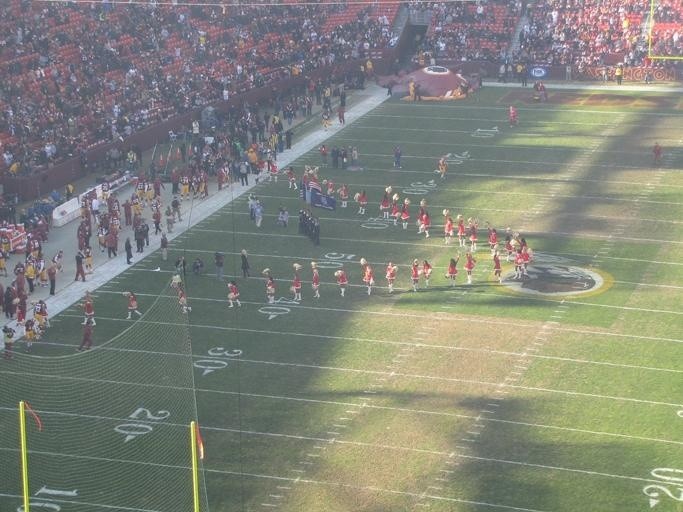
[48,170,136,226]
[1,0,281,180]
[282,0,682,85]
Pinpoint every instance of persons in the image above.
[0,0,683,359]
